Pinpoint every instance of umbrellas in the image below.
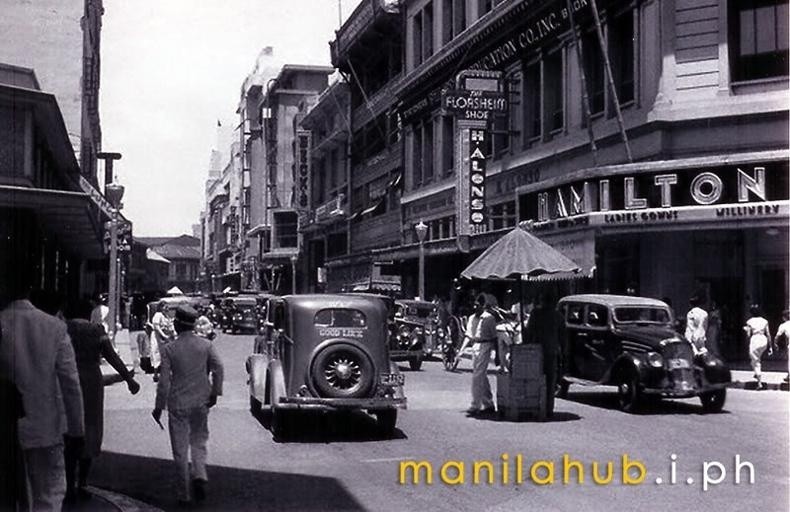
[456,222,582,342]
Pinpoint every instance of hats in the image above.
[175,305,198,326]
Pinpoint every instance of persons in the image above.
[0,256,88,512]
[151,301,177,381]
[53,283,141,503]
[135,286,153,375]
[91,286,112,338]
[151,304,225,510]
[447,292,789,414]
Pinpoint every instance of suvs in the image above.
[555,294,732,414]
[246,294,406,442]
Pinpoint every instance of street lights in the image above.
[290,254,298,295]
[415,221,428,302]
[104,175,124,358]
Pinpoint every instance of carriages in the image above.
[442,276,531,372]
